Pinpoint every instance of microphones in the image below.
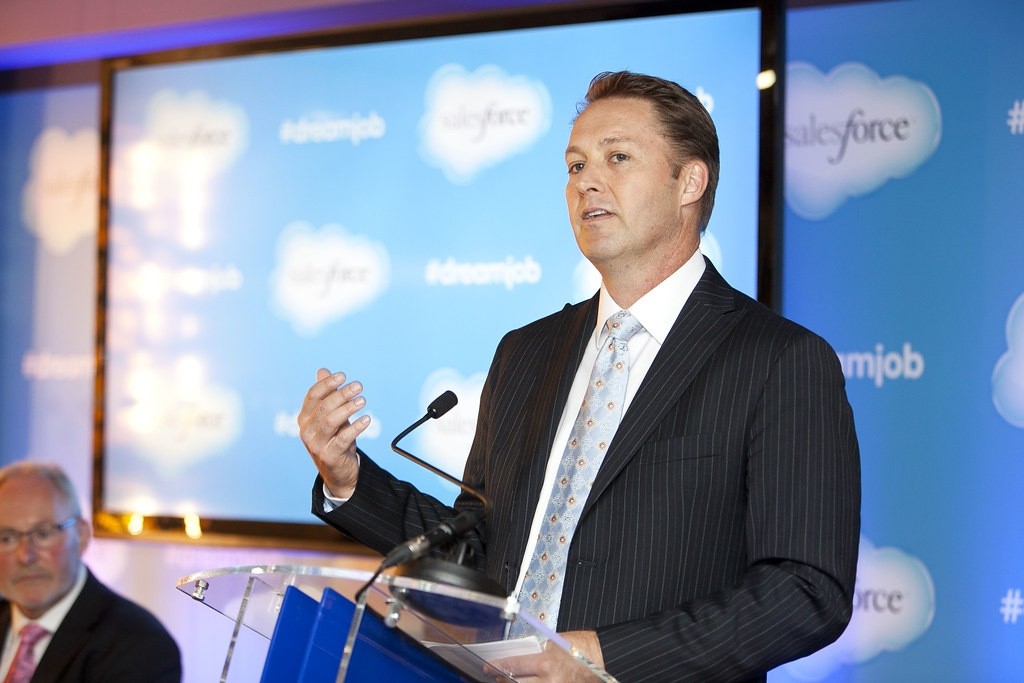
[380,390,492,567]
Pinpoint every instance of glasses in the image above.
[0,516,77,552]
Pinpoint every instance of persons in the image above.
[297,69,860,683]
[0,458,185,683]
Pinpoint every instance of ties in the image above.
[3,623,48,683]
[508,310,642,644]
